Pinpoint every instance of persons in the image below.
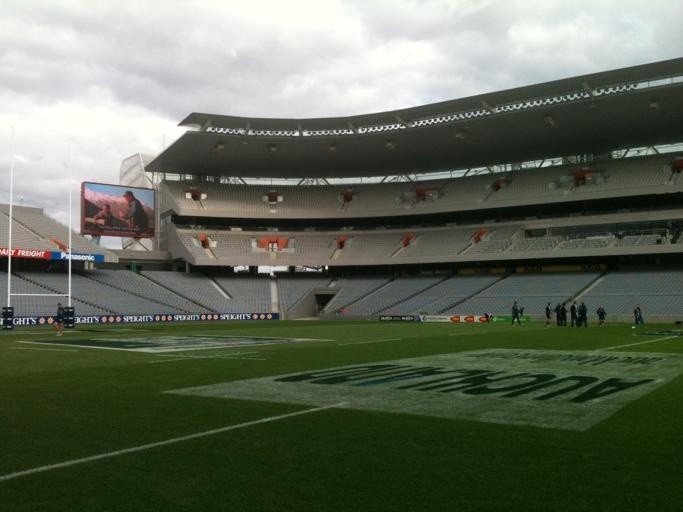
[520,307,525,316]
[634,304,644,327]
[596,303,607,326]
[511,303,523,326]
[54,304,65,337]
[94,205,114,231]
[120,190,149,233]
[544,301,588,327]
